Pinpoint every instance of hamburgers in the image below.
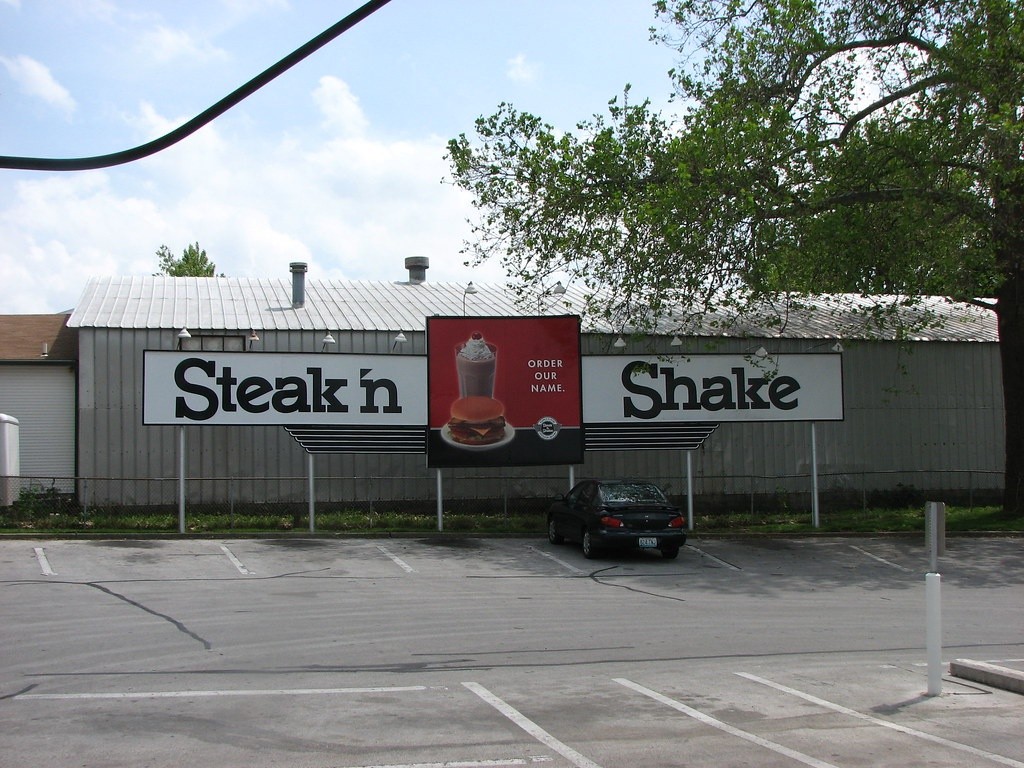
[448,397,507,444]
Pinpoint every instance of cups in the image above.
[455,342,497,399]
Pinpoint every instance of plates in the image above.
[441,421,515,451]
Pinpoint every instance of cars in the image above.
[546,478,688,559]
